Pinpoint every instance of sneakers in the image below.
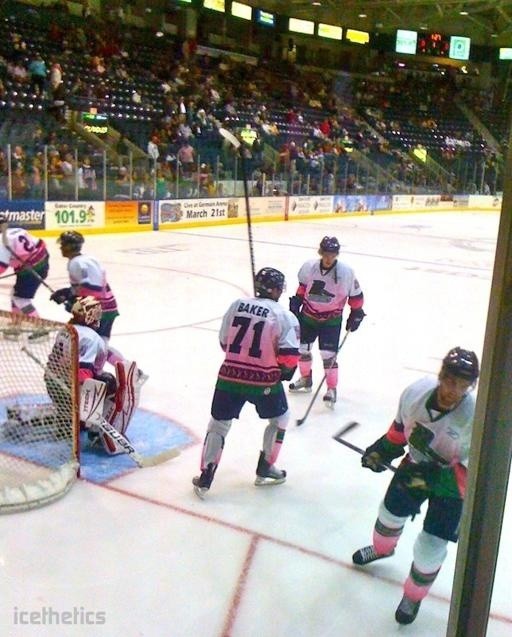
[353,545,394,564]
[2,329,19,336]
[29,329,48,339]
[290,377,312,388]
[395,594,421,625]
[323,389,336,402]
[256,460,286,479]
[192,474,213,489]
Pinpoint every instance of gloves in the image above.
[346,308,364,331]
[401,457,460,499]
[65,297,77,312]
[50,289,72,304]
[289,297,302,316]
[362,435,405,472]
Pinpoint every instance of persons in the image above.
[0,296,117,443]
[351,342,480,627]
[289,233,365,412]
[1,0,511,206]
[187,262,301,500]
[1,213,53,343]
[49,228,120,339]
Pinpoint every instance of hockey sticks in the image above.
[22,346,181,468]
[334,422,398,473]
[2,223,73,307]
[295,327,351,425]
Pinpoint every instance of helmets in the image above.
[254,267,284,295]
[442,346,479,382]
[73,295,103,328]
[60,231,84,254]
[320,236,340,252]
